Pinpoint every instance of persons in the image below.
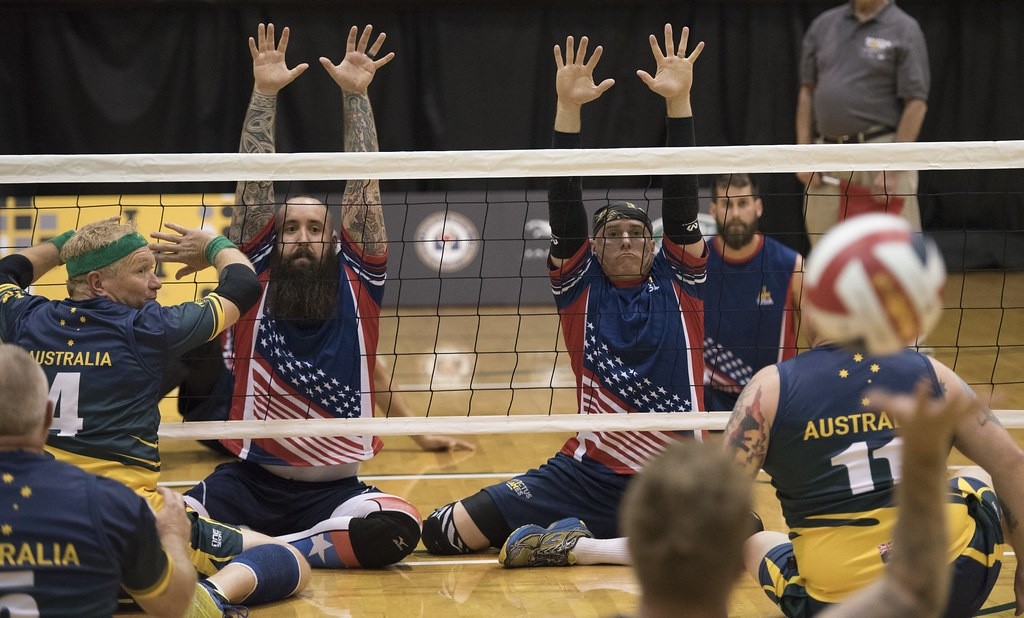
[796,0,930,246]
[1,215,311,618]
[181,23,423,571]
[720,309,1024,618]
[704,172,804,411]
[618,378,987,618]
[421,25,764,569]
[178,326,475,456]
[1,344,198,618]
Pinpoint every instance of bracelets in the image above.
[48,230,77,264]
[205,235,240,269]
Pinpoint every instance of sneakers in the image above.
[498,516,594,567]
[177,577,249,618]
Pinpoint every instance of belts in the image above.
[814,127,896,143]
